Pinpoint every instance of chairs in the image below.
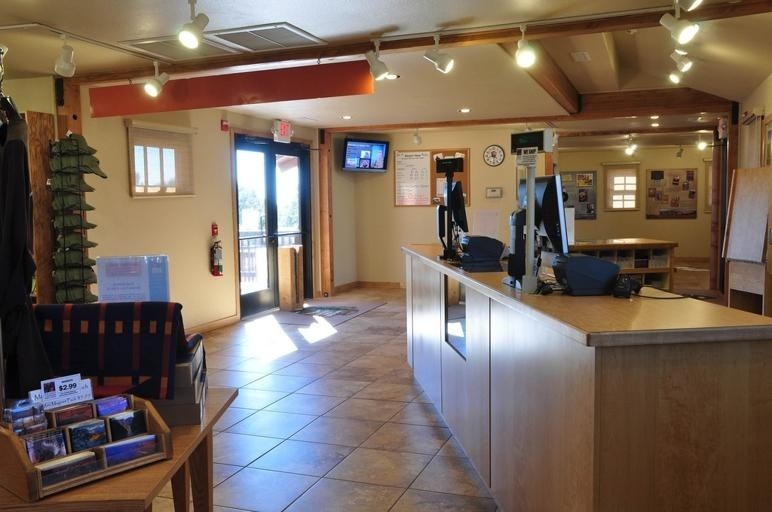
[31,299,211,425]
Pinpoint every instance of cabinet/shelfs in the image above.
[569,237,680,294]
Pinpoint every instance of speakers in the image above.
[319,129,325,145]
[54,78,64,106]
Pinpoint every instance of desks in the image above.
[0,386,239,512]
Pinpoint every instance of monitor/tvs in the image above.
[542,174,570,255]
[341,138,390,174]
[453,181,469,233]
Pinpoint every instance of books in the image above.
[3,395,159,486]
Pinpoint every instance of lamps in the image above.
[143,59,173,99]
[365,23,535,83]
[658,0,705,85]
[51,32,80,82]
[177,0,210,51]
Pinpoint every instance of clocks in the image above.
[482,144,505,167]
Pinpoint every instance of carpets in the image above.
[240,300,388,327]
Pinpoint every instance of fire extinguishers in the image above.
[210,240,223,276]
